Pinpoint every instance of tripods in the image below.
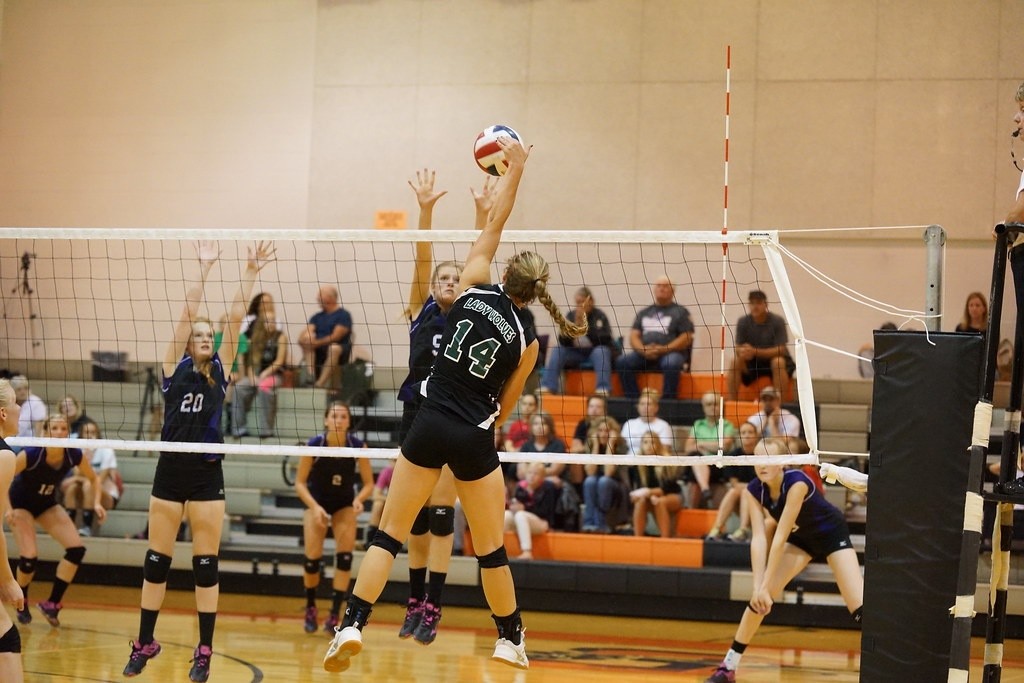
[133,366,165,459]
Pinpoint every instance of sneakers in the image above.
[15,597,32,624]
[491,624,528,670]
[398,596,430,639]
[304,606,318,633]
[188,639,212,682]
[325,611,338,638]
[413,600,442,646]
[37,601,61,628]
[323,623,364,674]
[703,665,738,683]
[123,640,163,677]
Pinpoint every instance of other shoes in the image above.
[707,527,722,541]
[700,491,715,509]
[728,528,747,542]
[583,524,610,535]
[536,385,553,395]
[77,526,92,539]
[595,389,610,398]
[516,551,532,560]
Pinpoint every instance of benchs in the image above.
[0,356,797,625]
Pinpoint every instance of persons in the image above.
[451,386,809,560]
[364,459,397,550]
[296,402,373,635]
[398,167,500,645]
[986,453,1024,538]
[324,136,589,672]
[132,512,189,542]
[214,293,287,437]
[535,278,695,399]
[956,292,989,336]
[5,414,108,627]
[992,85,1024,494]
[123,240,277,683]
[0,379,25,683]
[705,438,863,683]
[299,287,352,388]
[7,374,122,537]
[723,289,795,404]
[882,323,897,330]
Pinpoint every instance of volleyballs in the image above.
[474,125,525,177]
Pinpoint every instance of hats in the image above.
[760,387,781,399]
